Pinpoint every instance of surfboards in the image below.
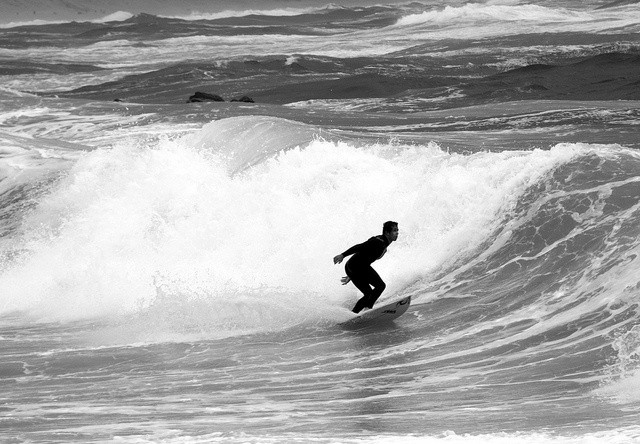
[335,295,412,330]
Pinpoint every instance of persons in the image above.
[333,221,398,312]
